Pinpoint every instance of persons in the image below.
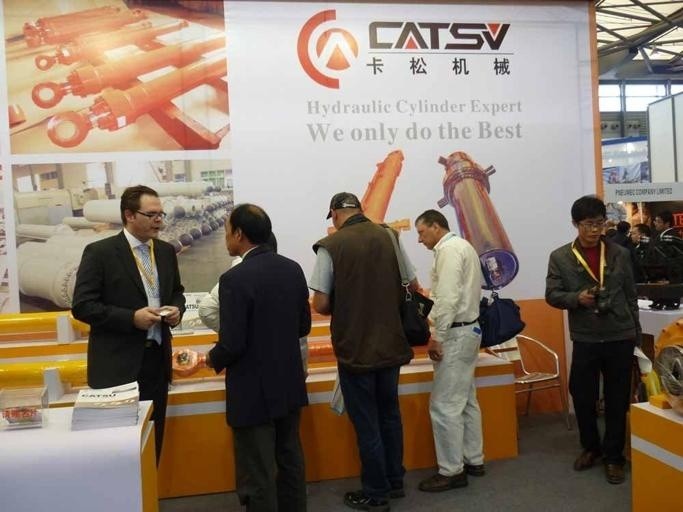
[605,203,682,284]
[415,209,486,492]
[306,192,419,512]
[71,185,186,471]
[206,203,312,510]
[544,195,642,484]
[198,231,277,334]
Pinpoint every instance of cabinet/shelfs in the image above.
[628,398,683,512]
[0,399,161,512]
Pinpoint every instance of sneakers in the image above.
[389,487,405,498]
[345,492,390,512]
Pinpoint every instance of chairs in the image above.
[486,334,572,440]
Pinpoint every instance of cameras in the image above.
[591,290,612,316]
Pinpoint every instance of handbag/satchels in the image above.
[632,357,646,401]
[385,228,434,347]
[479,261,525,348]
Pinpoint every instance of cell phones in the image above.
[154,308,172,318]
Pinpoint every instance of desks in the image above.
[636,299,683,347]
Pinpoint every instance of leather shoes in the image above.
[419,470,467,492]
[465,464,484,476]
[607,463,625,483]
[574,450,603,471]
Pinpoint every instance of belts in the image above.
[449,320,476,328]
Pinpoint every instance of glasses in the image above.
[578,222,606,231]
[137,210,167,221]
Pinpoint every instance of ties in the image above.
[140,245,158,297]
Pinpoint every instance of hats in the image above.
[326,192,361,219]
